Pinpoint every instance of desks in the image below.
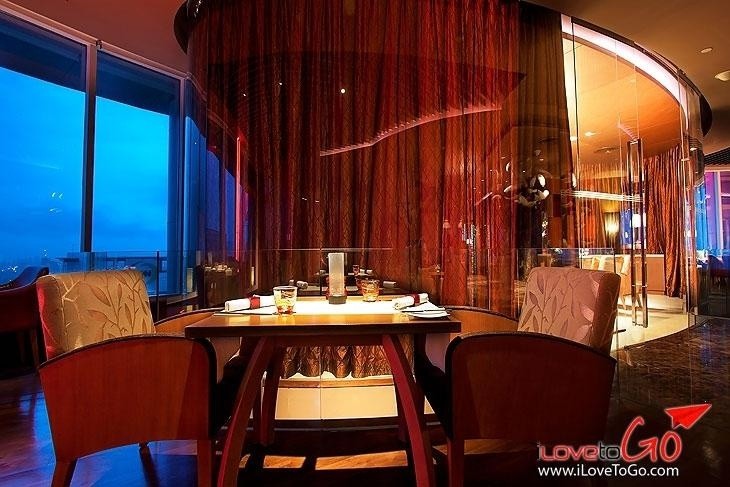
[182,269,463,486]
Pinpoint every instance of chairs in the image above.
[0,266,51,369]
[411,262,626,486]
[31,265,243,486]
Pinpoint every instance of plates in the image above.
[407,308,451,320]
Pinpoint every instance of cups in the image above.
[319,270,327,275]
[353,264,359,275]
[355,276,368,289]
[383,281,396,288]
[367,269,374,274]
[360,279,381,303]
[360,268,365,275]
[273,285,297,315]
